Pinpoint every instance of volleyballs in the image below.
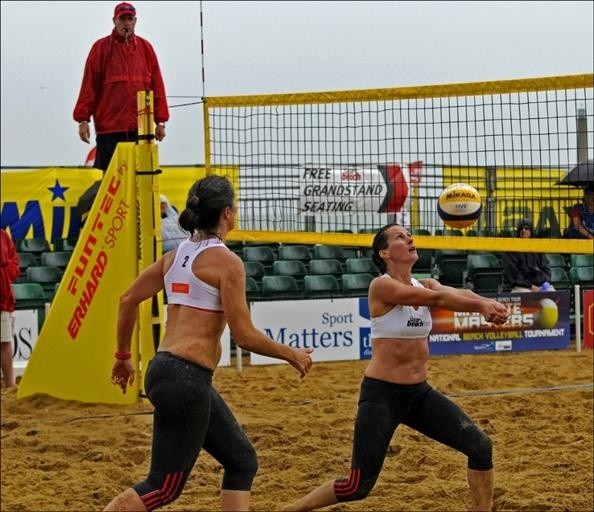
[438,182,482,228]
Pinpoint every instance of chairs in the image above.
[0,226,594,308]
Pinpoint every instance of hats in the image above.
[113,3,136,18]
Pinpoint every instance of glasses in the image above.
[119,6,136,11]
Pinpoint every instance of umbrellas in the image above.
[554,157,593,187]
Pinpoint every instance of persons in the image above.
[566,187,593,240]
[267,219,513,511]
[157,193,188,251]
[1,229,24,392]
[101,172,320,511]
[501,216,558,293]
[71,0,169,169]
